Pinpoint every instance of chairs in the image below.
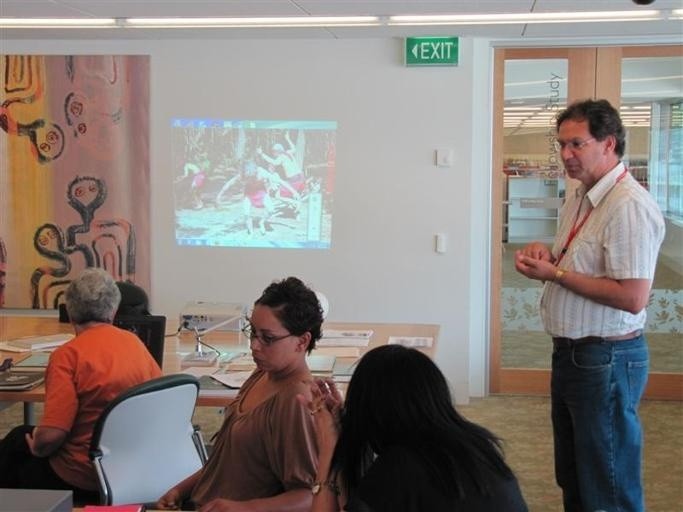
[88,372,209,507]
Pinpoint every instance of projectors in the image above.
[178,300,248,332]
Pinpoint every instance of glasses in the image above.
[554,138,606,149]
[242,324,312,344]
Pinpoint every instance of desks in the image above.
[0,314,440,426]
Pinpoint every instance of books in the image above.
[0,332,76,391]
[181,328,432,393]
[82,506,142,512]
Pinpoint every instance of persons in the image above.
[180,129,320,235]
[296,344,530,511]
[513,95,666,512]
[0,267,165,506]
[151,276,323,511]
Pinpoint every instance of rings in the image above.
[316,405,325,409]
[309,408,317,415]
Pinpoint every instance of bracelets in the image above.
[309,479,328,495]
[555,268,566,285]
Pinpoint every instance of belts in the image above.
[553,329,641,342]
